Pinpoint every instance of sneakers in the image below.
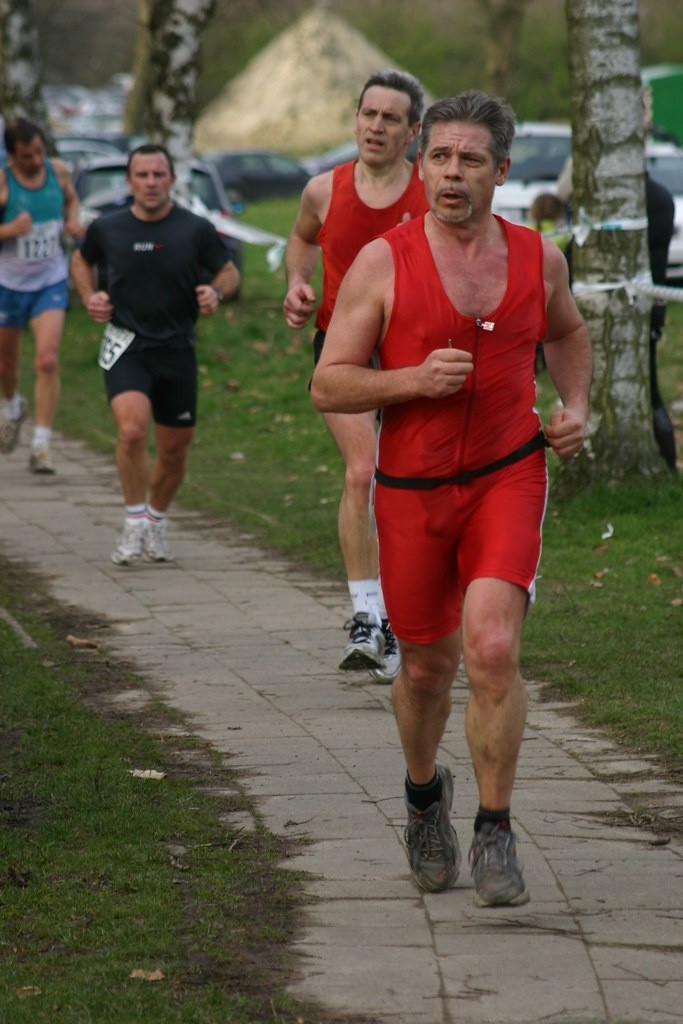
[467,826,531,909]
[338,611,388,670]
[29,451,54,474]
[367,608,402,684]
[142,519,173,563]
[404,764,460,893]
[0,394,26,455]
[112,524,146,566]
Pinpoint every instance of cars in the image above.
[43,77,423,271]
[486,122,683,292]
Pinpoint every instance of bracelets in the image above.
[215,287,224,302]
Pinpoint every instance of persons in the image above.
[0,117,83,476]
[284,69,432,684]
[70,143,242,565]
[562,162,676,472]
[308,93,595,906]
